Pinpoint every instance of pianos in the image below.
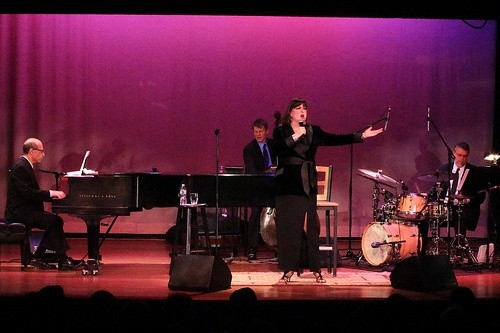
[49,170,277,277]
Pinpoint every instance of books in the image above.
[475,244,494,263]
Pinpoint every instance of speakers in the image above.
[389,255,458,293]
[168,254,233,292]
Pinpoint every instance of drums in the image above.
[426,202,452,219]
[396,192,428,221]
[361,221,423,267]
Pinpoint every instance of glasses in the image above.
[32,147,45,153]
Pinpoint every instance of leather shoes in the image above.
[29,259,56,270]
[247,248,256,259]
[58,257,85,271]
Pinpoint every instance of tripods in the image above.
[423,175,478,267]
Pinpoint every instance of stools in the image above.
[0,222,31,271]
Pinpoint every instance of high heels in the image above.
[279,271,294,283]
[313,269,326,283]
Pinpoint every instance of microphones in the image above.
[299,120,305,139]
[427,107,430,131]
[383,107,391,131]
[371,242,380,248]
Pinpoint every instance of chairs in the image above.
[316,165,339,277]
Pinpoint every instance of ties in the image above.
[263,144,270,168]
[451,168,460,196]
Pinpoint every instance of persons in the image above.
[5,138,84,271]
[273,99,384,283]
[244,118,276,260]
[418,141,486,262]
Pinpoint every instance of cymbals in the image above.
[355,167,400,189]
[417,172,457,182]
[453,194,468,201]
